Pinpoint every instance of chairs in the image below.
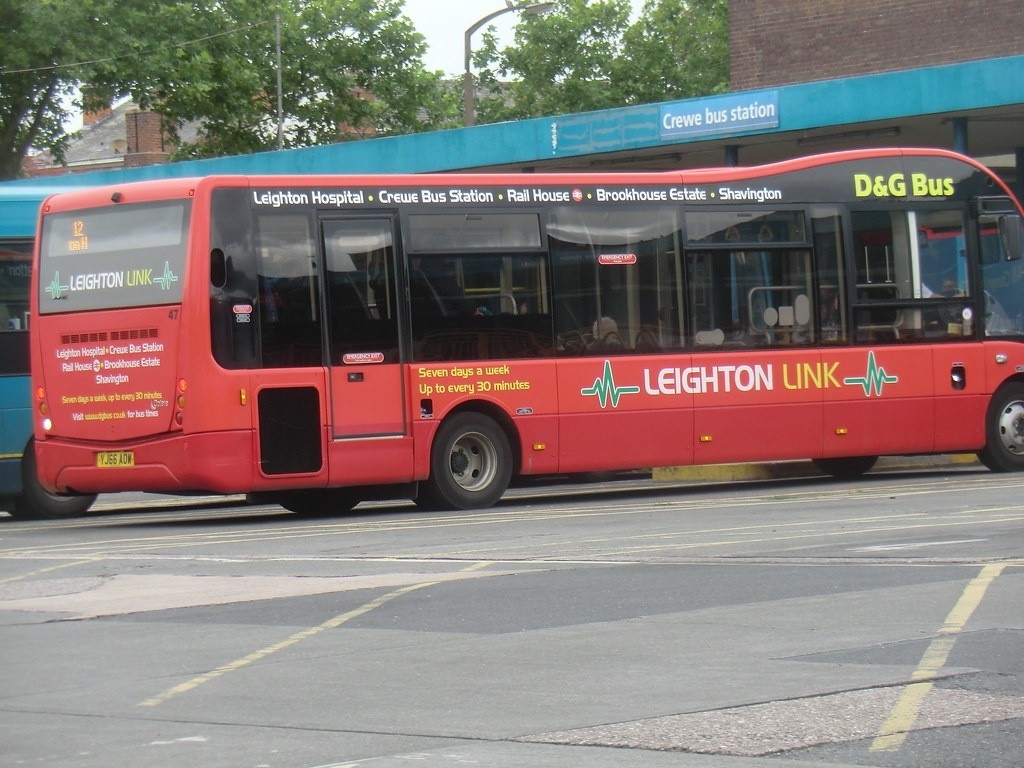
[346,268,664,353]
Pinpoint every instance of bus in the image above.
[29,148,1024,517]
[0,183,771,522]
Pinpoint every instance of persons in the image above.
[920,282,947,300]
[943,279,955,291]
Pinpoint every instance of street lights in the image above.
[463,0,555,128]
